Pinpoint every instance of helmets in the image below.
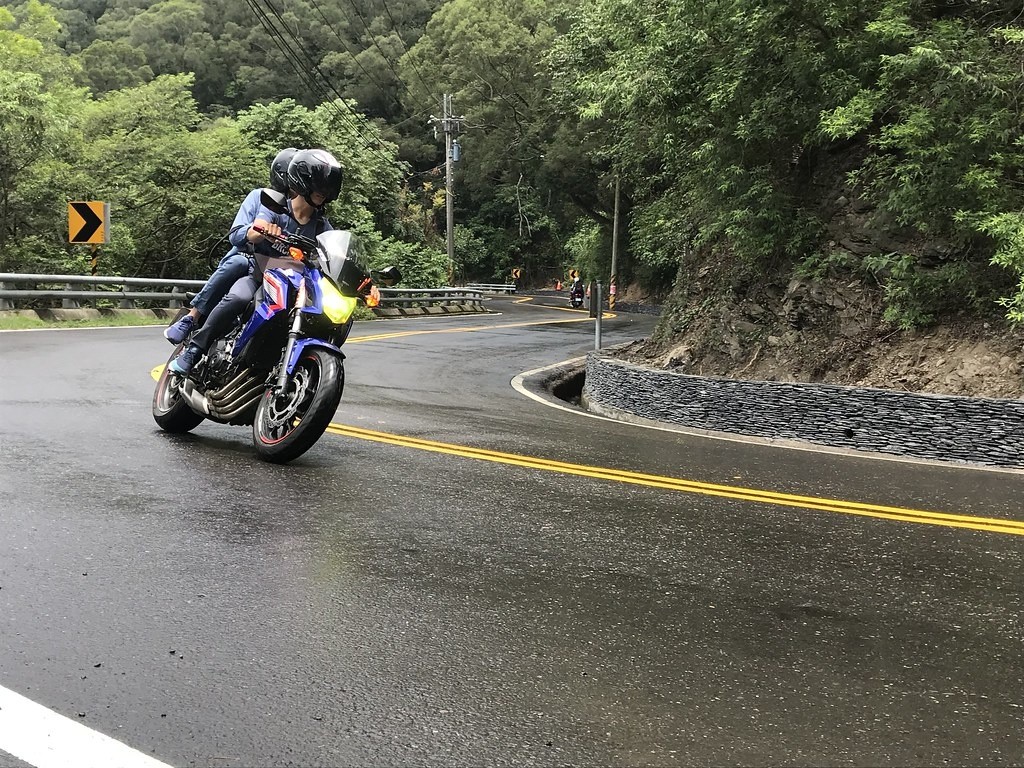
[270,148,303,192]
[287,148,342,203]
[574,277,579,283]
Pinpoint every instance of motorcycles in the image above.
[569,290,583,308]
[151,223,404,463]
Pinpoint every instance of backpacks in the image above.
[576,281,582,293]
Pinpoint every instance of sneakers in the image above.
[164,314,199,344]
[168,343,202,373]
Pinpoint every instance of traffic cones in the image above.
[556,280,562,291]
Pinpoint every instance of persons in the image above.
[163,146,380,376]
[569,277,584,306]
